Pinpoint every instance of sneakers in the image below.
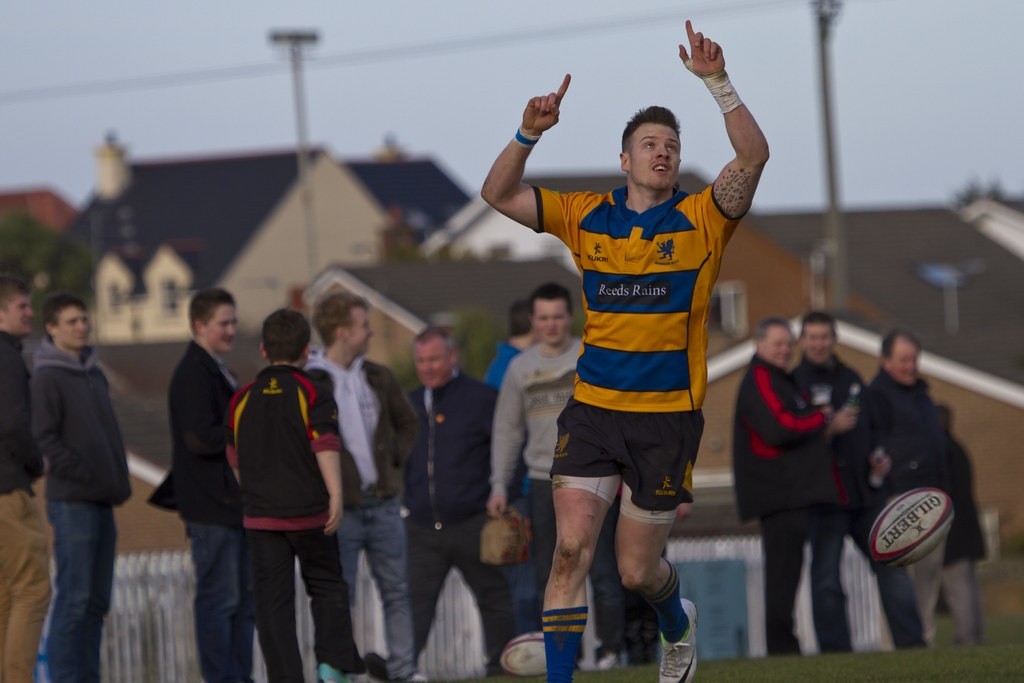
[658,598,697,683]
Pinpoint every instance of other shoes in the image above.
[317,661,385,683]
[595,652,622,671]
[404,671,428,682]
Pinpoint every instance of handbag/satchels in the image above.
[480,512,530,566]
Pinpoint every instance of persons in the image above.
[223,309,365,683]
[481,18,769,683]
[0,277,51,683]
[731,311,988,657]
[482,280,693,673]
[362,326,516,683]
[28,295,132,683]
[303,290,428,683]
[146,289,252,683]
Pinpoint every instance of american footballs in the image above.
[867,490,956,569]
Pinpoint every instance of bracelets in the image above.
[513,129,541,149]
